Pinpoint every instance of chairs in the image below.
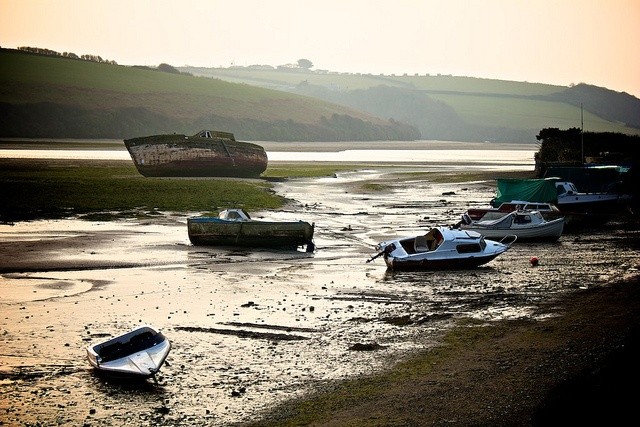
[99,341,126,362]
[414,235,429,253]
[130,330,155,353]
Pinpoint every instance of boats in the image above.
[366,226,518,269]
[463,199,573,228]
[85,325,171,379]
[187,208,316,252]
[489,175,634,227]
[456,209,566,243]
[125,129,268,177]
